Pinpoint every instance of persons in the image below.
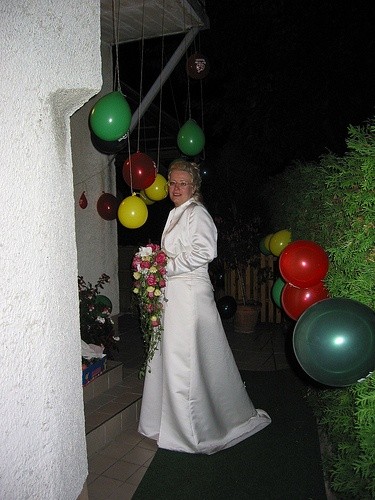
[137,160,272,455]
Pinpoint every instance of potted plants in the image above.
[209,203,263,335]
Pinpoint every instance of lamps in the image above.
[188,5,211,81]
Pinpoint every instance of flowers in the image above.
[78,272,124,356]
[130,244,168,382]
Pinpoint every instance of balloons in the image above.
[89,91,131,142]
[80,153,170,229]
[219,229,375,387]
[177,119,205,156]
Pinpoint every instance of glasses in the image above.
[165,180,191,187]
